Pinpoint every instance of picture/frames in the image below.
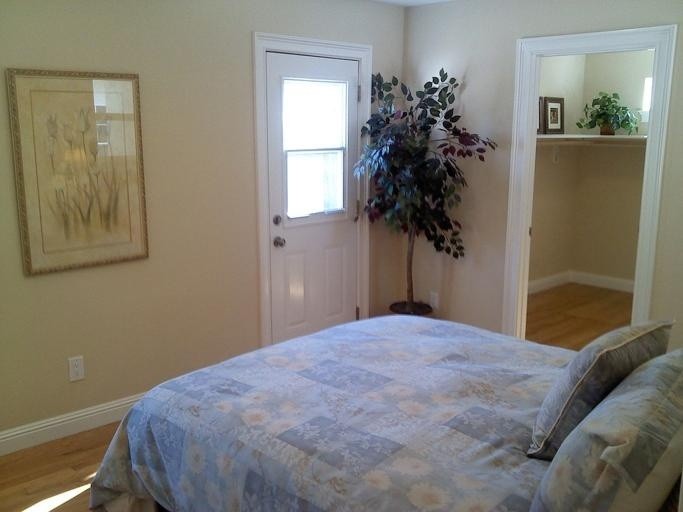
[4,67,152,279]
[535,95,565,136]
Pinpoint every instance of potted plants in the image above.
[574,90,641,136]
[350,66,499,318]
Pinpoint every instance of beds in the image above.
[127,313,682,511]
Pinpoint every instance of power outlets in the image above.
[428,289,440,311]
[66,355,86,383]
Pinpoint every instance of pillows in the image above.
[522,320,682,511]
